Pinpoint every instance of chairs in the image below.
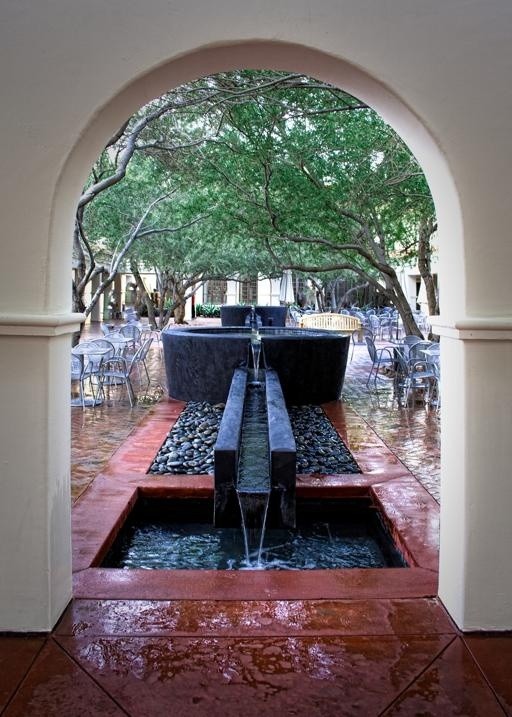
[288,303,441,426]
[71,306,161,413]
